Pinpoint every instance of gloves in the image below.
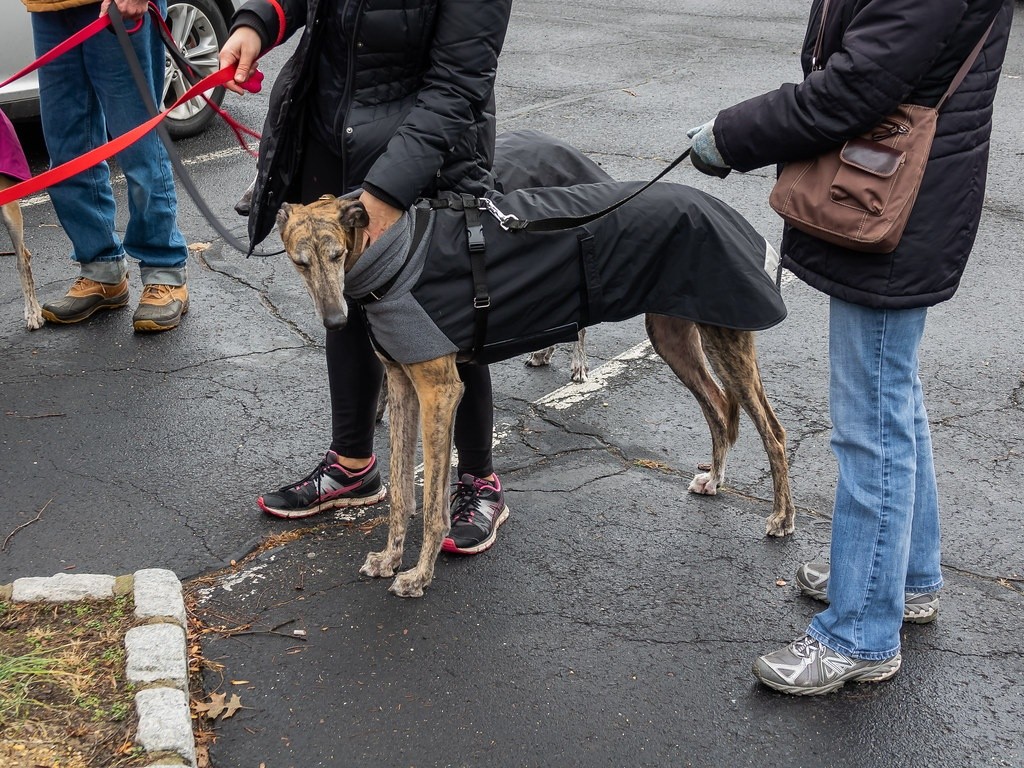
[686,117,732,179]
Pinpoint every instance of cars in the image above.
[0,0,245,140]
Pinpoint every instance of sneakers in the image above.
[796,564,939,623]
[131,284,189,330]
[441,472,509,554]
[257,450,388,518]
[42,275,130,324]
[752,633,902,695]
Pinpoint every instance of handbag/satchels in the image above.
[768,66,939,253]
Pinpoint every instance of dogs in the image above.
[1,105,45,334]
[234,124,797,599]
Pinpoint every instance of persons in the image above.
[215,0,511,552]
[22,0,190,333]
[687,0,1016,695]
